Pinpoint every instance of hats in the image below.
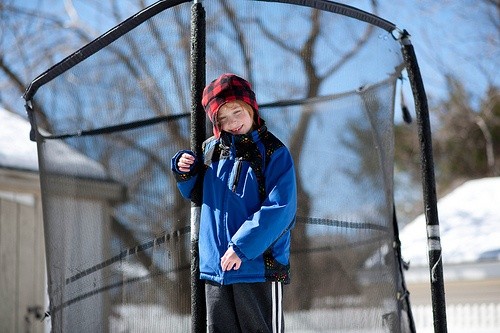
[199,72,263,138]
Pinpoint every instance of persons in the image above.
[170,71,297,333]
